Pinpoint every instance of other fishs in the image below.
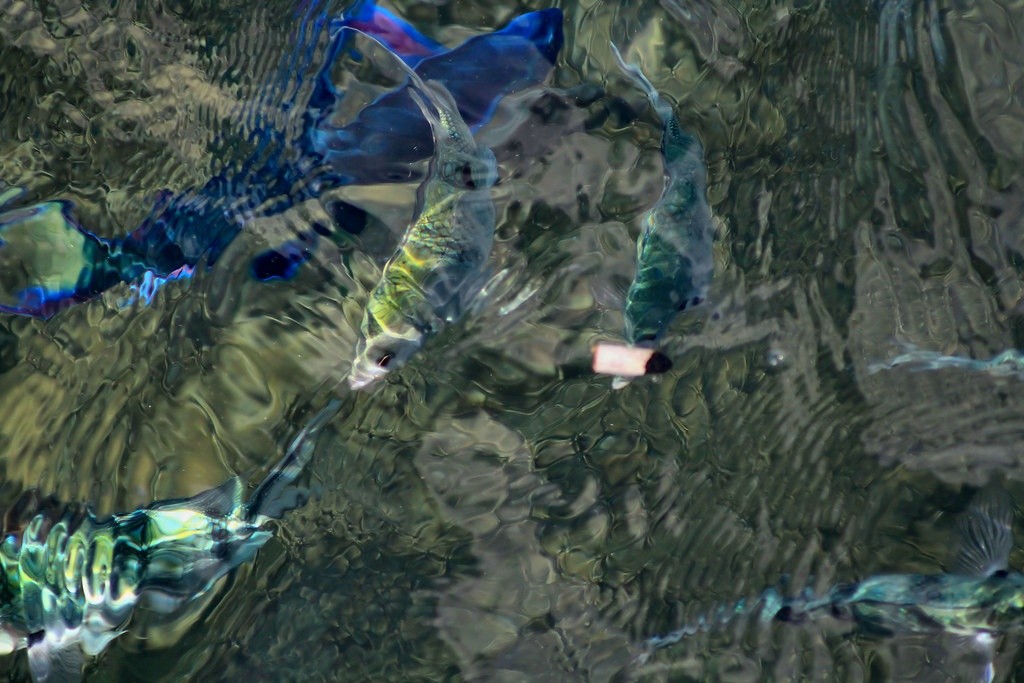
[607,41,713,387]
[249,232,318,283]
[250,3,566,218]
[626,495,1024,683]
[332,25,499,388]
[0,476,281,683]
